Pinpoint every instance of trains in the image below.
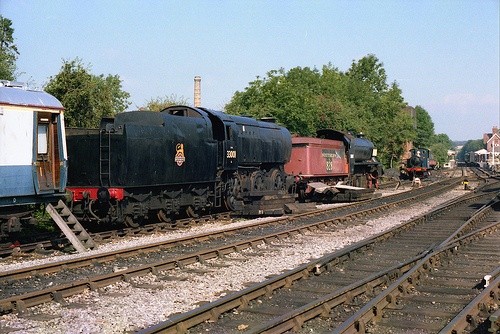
[399,147,432,181]
[0,79,384,250]
